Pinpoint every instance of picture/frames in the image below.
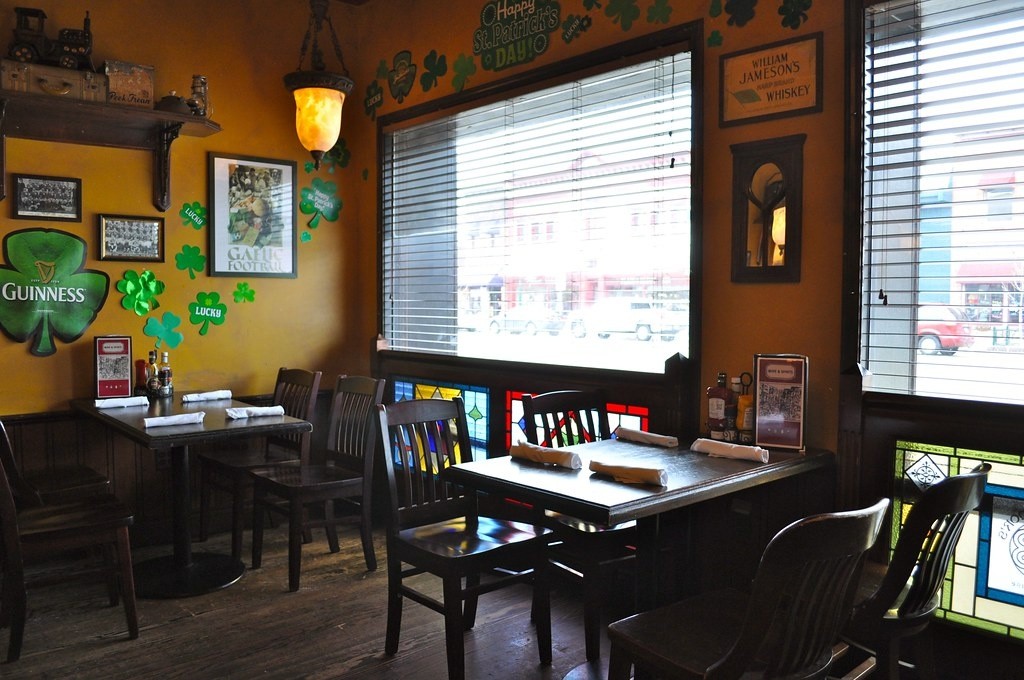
[208,150,298,278]
[14,173,82,222]
[98,213,165,263]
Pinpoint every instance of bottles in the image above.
[134,349,173,396]
[191,75,207,116]
[706,371,754,443]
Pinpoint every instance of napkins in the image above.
[144,411,206,428]
[225,404,284,420]
[182,390,232,403]
[94,395,149,409]
[510,425,770,486]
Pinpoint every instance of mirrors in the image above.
[730,132,808,283]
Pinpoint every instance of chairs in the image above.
[372,397,554,680]
[751,462,992,680]
[522,386,679,661]
[249,375,386,590]
[0,461,138,662]
[0,420,111,563]
[606,498,889,680]
[193,368,322,558]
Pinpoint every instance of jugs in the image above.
[154,90,192,115]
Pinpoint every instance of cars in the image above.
[915,301,976,357]
[456,278,690,341]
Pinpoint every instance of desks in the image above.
[436,433,835,680]
[64,392,313,599]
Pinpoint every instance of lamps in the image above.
[282,0,355,171]
[772,201,786,256]
